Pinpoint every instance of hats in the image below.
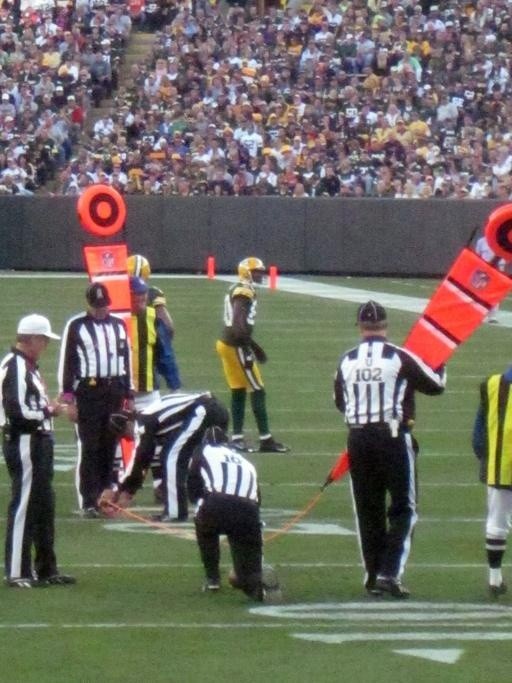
[202,424,230,447]
[130,275,147,294]
[86,282,113,308]
[355,301,386,325]
[106,411,127,439]
[17,313,62,340]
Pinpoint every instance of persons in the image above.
[0,314,77,589]
[216,257,291,453]
[130,277,180,396]
[185,426,263,601]
[109,393,229,523]
[58,282,134,519]
[471,370,512,598]
[332,301,446,600]
[127,254,175,339]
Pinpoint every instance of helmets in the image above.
[238,257,266,288]
[127,254,150,282]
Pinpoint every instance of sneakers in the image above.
[11,578,36,589]
[260,435,291,452]
[230,435,257,453]
[376,573,410,601]
[149,513,170,522]
[204,581,221,595]
[365,588,381,599]
[488,581,506,600]
[234,580,264,602]
[40,574,75,585]
